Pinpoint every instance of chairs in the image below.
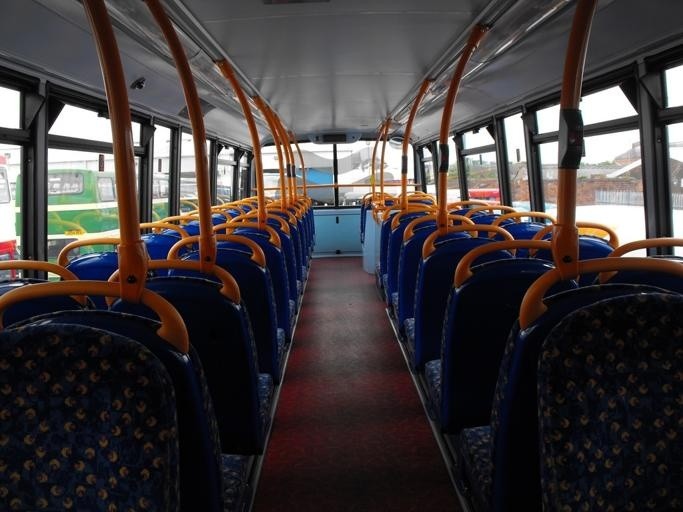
[361,192,683,512]
[1,196,315,512]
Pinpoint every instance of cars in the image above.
[312,191,394,206]
[467,182,500,202]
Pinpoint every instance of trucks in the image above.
[0,156,18,290]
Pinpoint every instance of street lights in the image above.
[366,141,371,194]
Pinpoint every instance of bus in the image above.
[14,169,232,288]
